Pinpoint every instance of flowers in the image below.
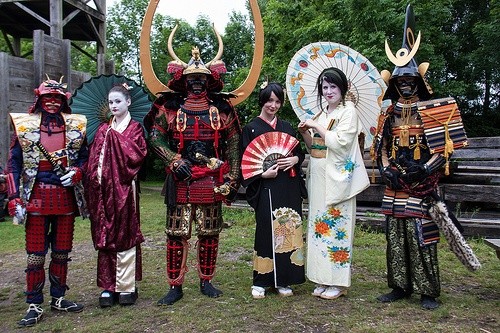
[260,82,269,89]
[123,82,133,91]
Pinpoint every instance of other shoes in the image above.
[200,278,223,297]
[420,294,439,309]
[50,296,84,312]
[377,289,411,303]
[18,303,43,327]
[157,285,183,305]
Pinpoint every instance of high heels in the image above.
[99,290,114,307]
[118,287,138,304]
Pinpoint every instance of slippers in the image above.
[312,285,328,296]
[321,287,348,299]
[251,286,265,298]
[271,286,293,297]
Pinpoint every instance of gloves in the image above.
[379,166,407,190]
[402,161,431,184]
[8,191,27,224]
[169,153,193,182]
[59,166,84,187]
[224,180,240,203]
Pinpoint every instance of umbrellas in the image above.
[286,40,387,151]
[68,73,153,148]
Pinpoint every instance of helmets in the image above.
[381,4,434,101]
[167,22,226,93]
[29,73,71,115]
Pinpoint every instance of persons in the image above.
[80,83,147,307]
[6,73,89,328]
[149,67,242,305]
[375,63,454,308]
[238,82,307,299]
[297,68,370,299]
